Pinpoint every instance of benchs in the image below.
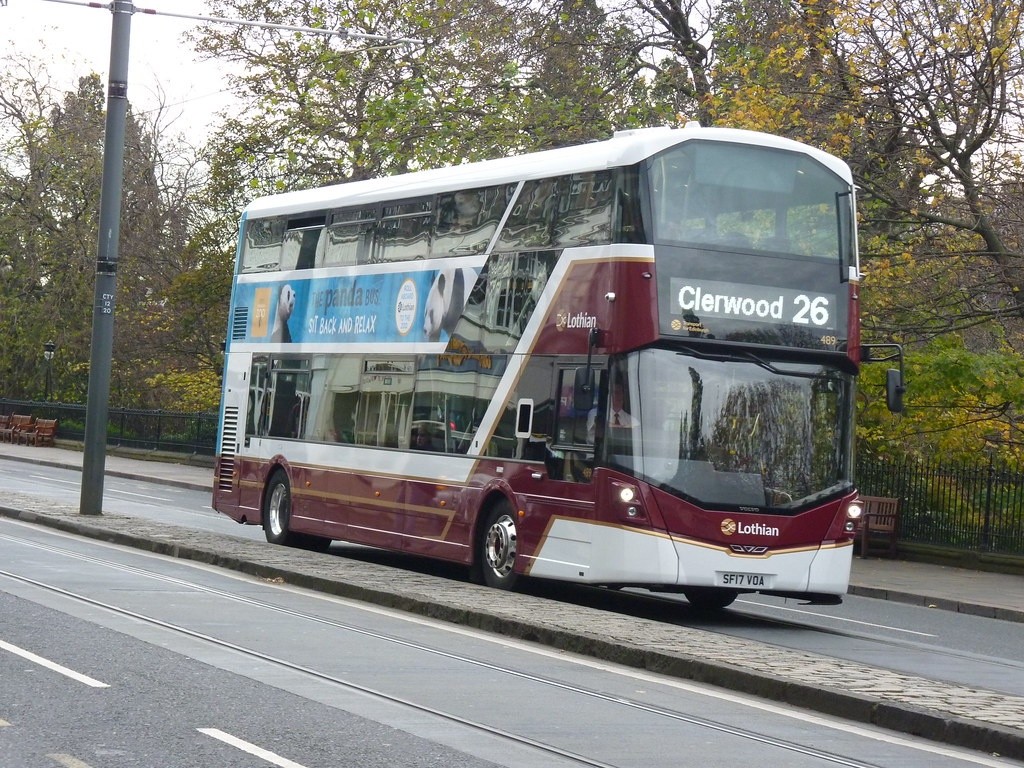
[0,412,57,448]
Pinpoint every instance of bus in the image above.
[212,120,908,609]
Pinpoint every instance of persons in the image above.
[410,423,435,450]
[585,380,643,460]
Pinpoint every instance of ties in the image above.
[614,414,620,425]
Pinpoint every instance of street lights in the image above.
[43,340,56,402]
